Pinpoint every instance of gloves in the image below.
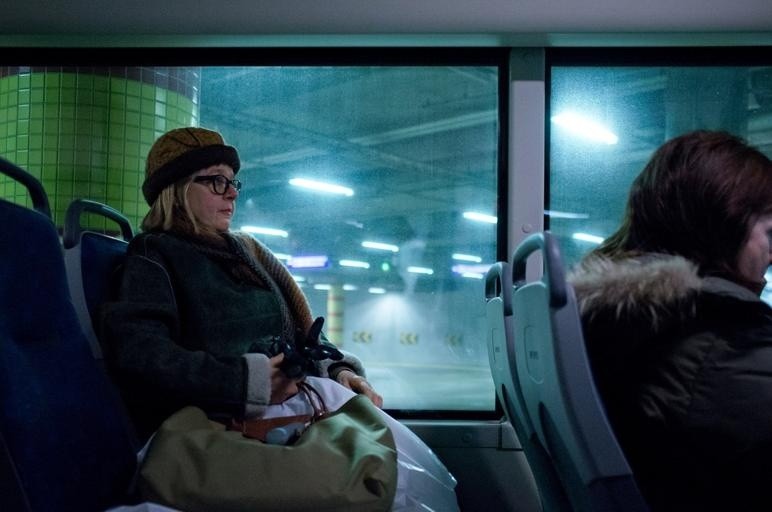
[277,317,343,374]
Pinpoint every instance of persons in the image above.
[577,130,772,510]
[95,127,384,438]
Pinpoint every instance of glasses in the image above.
[194,174,242,195]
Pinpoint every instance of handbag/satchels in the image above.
[140,382,398,512]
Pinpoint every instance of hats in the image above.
[142,128,240,208]
